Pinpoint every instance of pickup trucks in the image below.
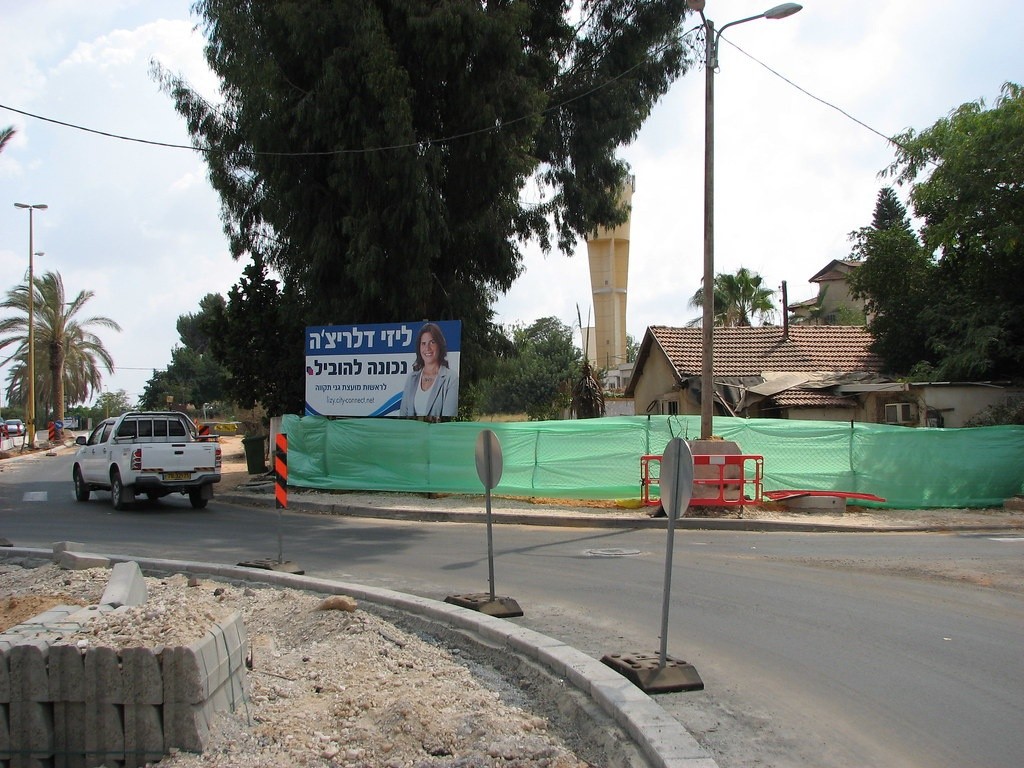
[72,410,222,513]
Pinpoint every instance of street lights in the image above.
[685,0,803,441]
[104,385,108,419]
[13,202,48,448]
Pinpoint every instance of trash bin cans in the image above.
[241,435,269,475]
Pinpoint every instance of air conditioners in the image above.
[885,403,918,424]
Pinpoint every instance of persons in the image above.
[399,323,458,416]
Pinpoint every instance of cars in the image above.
[2,420,26,437]
